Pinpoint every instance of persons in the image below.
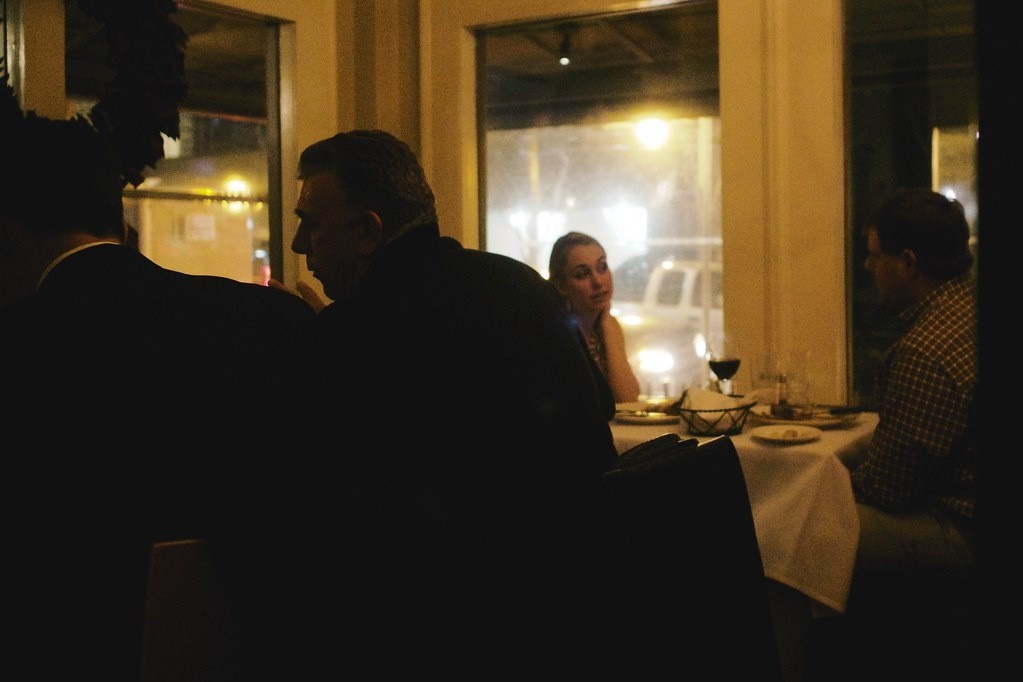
[1,0,627,682]
[550,231,640,403]
[846,186,978,562]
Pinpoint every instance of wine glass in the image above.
[708,337,740,396]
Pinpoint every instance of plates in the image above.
[750,424,823,444]
[757,407,860,427]
[612,410,682,423]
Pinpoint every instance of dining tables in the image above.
[610,412,880,613]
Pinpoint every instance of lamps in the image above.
[556,22,579,67]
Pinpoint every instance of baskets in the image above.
[674,394,757,436]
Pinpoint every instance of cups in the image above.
[749,354,778,408]
[786,348,810,406]
